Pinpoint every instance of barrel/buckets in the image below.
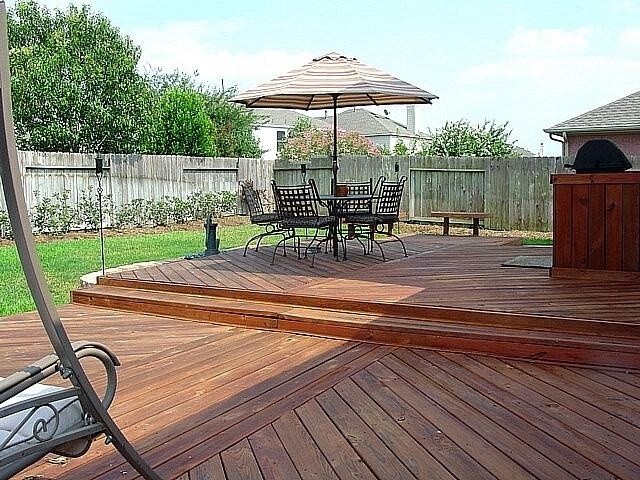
[336,183,348,197]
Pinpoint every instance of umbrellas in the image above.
[227,52,439,257]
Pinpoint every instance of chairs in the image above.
[238,175,407,269]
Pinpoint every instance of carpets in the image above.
[501,255,551,268]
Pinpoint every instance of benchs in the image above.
[430,211,491,237]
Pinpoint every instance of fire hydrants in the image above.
[204,216,220,255]
